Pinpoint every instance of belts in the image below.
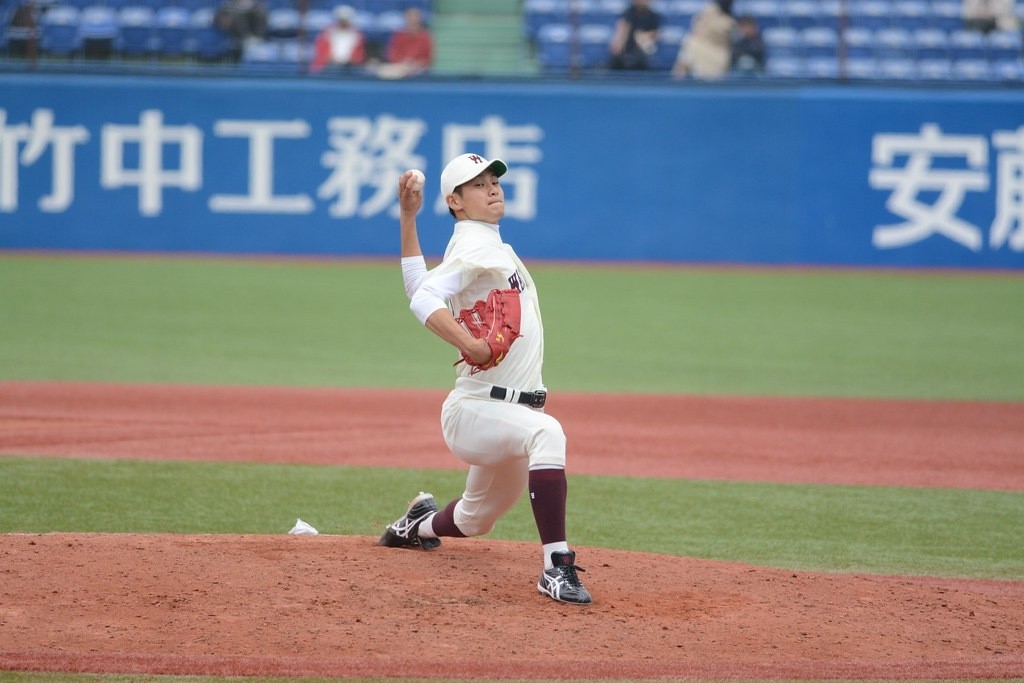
[490,386,546,408]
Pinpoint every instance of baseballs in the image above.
[406,168,426,193]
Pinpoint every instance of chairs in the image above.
[0,0,1024,87]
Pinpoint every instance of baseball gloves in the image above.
[449,287,523,379]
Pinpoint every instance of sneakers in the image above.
[378,491,442,551]
[536,550,592,604]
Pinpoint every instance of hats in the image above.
[335,4,354,21]
[441,153,507,201]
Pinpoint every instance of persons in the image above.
[670,0,738,82]
[961,0,1019,31]
[369,6,433,79]
[311,5,366,74]
[732,14,766,74]
[375,153,592,606]
[609,0,661,70]
